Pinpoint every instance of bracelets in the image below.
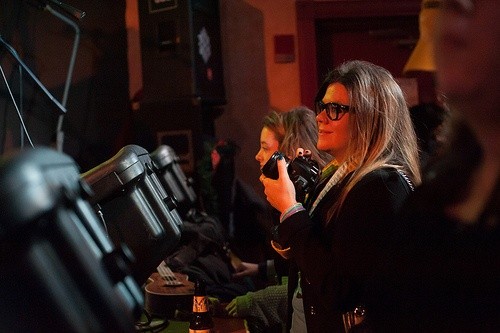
[280,200,306,223]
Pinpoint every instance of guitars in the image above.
[145,260,195,314]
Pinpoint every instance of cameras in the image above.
[262,151,321,196]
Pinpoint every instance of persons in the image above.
[376,1,500,333]
[211,147,288,299]
[225,107,338,333]
[259,60,422,333]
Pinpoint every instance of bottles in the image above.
[188,279,215,333]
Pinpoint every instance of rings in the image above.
[261,176,267,182]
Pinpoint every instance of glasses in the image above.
[318,100,352,120]
